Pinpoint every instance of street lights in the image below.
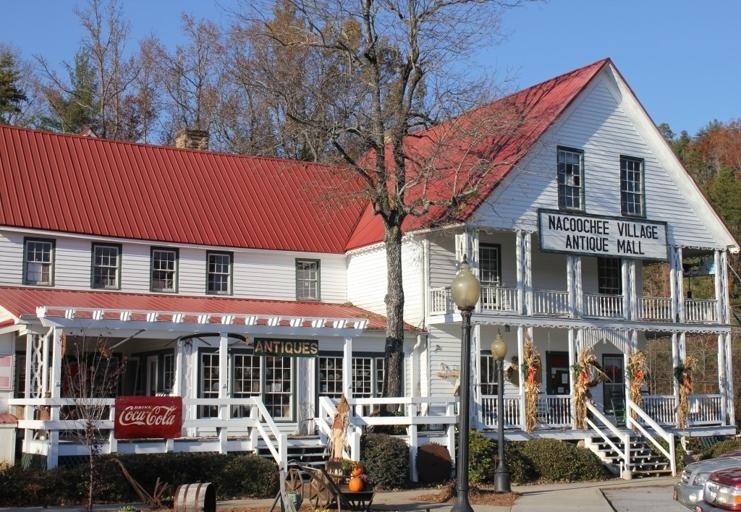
[449,250,482,512]
[490,326,511,496]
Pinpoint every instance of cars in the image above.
[670,449,740,512]
